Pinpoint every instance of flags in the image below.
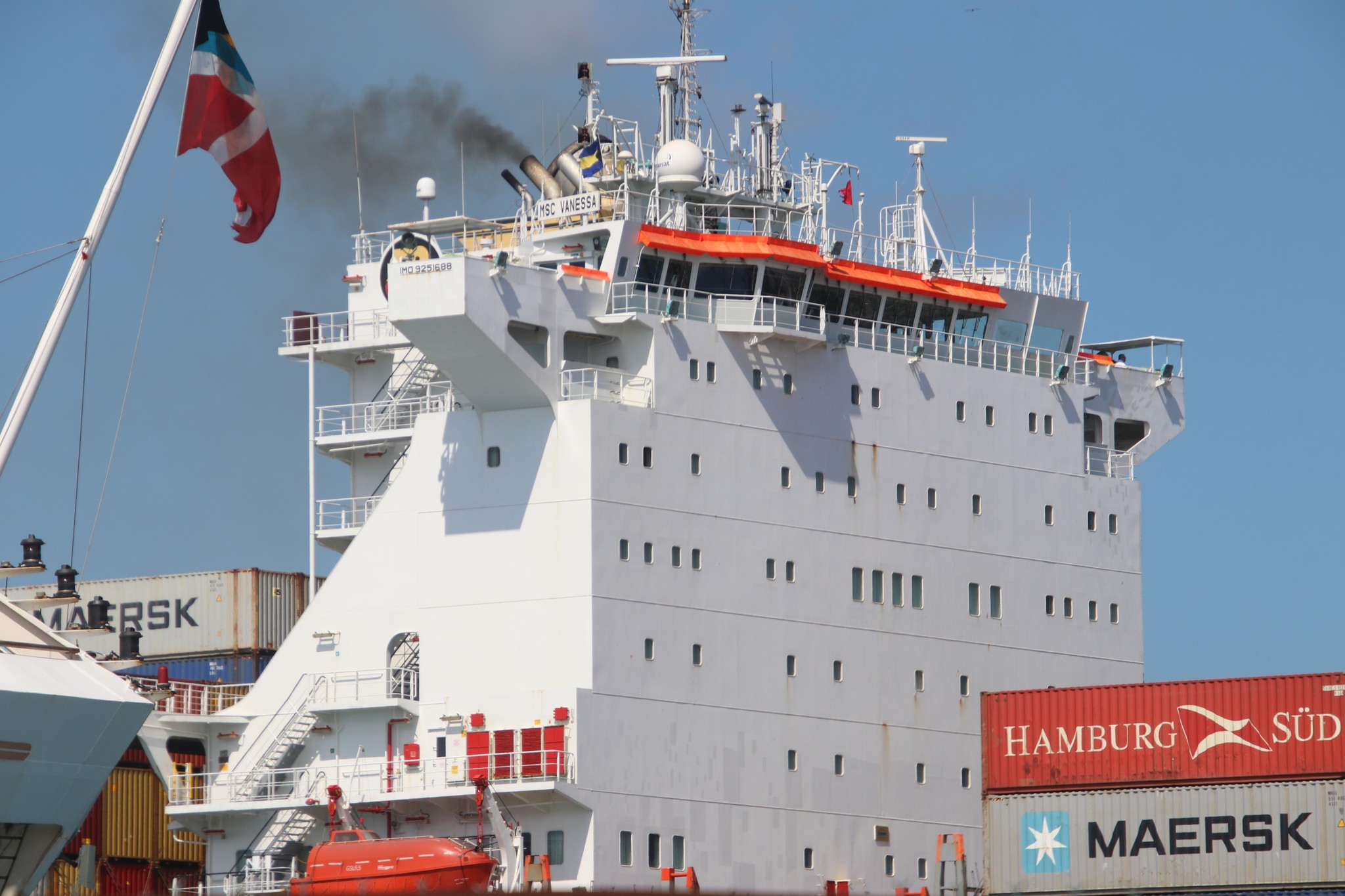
[839,178,853,207]
[176,0,282,244]
[578,135,604,178]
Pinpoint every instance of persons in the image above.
[1114,353,1127,369]
